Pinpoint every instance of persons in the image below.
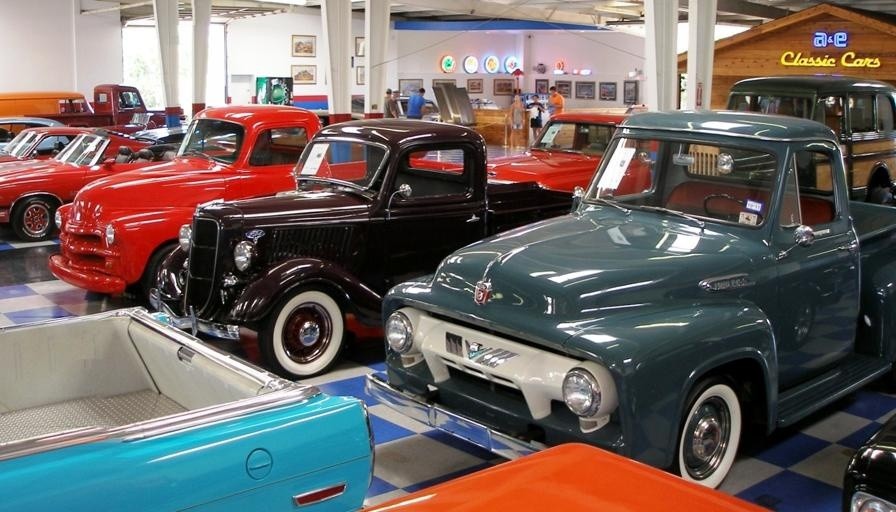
[546,86,564,149]
[868,180,896,207]
[525,95,544,146]
[382,88,427,120]
[510,95,524,149]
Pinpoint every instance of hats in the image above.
[386,88,392,93]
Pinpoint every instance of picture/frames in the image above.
[288,63,317,85]
[290,33,318,57]
[397,78,638,104]
[355,65,365,86]
[354,37,365,58]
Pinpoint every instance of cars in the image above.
[346,440,775,512]
[835,407,896,512]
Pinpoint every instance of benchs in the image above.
[666,182,835,228]
[387,168,463,199]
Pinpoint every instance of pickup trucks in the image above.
[378,104,894,488]
[0,305,379,512]
